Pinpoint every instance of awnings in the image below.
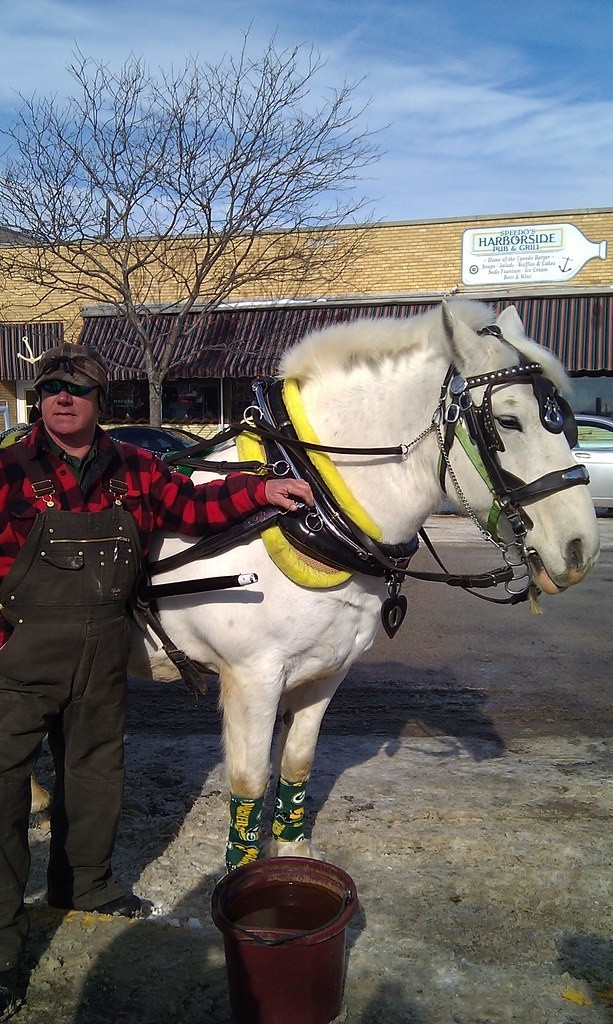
[0,323,63,381]
[78,295,613,382]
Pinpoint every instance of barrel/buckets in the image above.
[210,857,360,1024]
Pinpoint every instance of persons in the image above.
[0,343,316,1022]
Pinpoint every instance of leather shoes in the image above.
[82,893,143,918]
[0,963,27,1022]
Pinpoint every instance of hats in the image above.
[34,342,109,403]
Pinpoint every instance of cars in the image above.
[573,414,613,511]
[106,423,207,459]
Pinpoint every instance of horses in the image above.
[115,298,601,874]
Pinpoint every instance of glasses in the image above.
[40,378,97,396]
